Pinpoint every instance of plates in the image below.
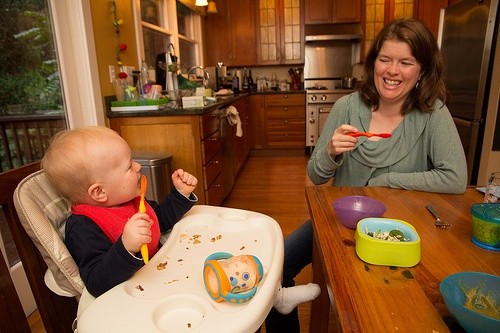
[476,186,500,198]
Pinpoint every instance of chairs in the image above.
[0,160,285,333]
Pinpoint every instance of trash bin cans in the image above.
[130,151,173,204]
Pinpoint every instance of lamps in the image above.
[195,0,217,13]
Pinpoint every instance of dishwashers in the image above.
[218,103,235,196]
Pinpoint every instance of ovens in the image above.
[305,103,335,146]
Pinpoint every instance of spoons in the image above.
[347,132,391,138]
[137,174,149,265]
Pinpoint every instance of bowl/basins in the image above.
[440,271,500,333]
[333,195,386,230]
[354,217,424,268]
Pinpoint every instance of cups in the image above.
[291,76,302,91]
[112,78,126,101]
[142,84,162,100]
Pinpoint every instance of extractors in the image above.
[304,24,364,44]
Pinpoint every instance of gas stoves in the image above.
[304,76,362,104]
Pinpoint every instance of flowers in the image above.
[113,0,128,79]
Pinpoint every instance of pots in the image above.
[342,77,357,89]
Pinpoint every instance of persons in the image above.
[42,125,320,314]
[264,19,468,333]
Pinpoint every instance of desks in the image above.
[305,185,500,333]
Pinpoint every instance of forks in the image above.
[425,204,450,226]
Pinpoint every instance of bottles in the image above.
[242,68,254,91]
[271,72,278,90]
[202,252,269,304]
[141,57,150,86]
[123,86,138,102]
[232,71,239,97]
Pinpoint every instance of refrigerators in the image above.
[434,0,500,186]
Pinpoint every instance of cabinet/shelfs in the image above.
[303,0,362,23]
[250,94,306,156]
[205,0,305,66]
[110,96,250,206]
[362,0,449,65]
[90,0,203,108]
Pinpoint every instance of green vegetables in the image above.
[367,229,405,241]
